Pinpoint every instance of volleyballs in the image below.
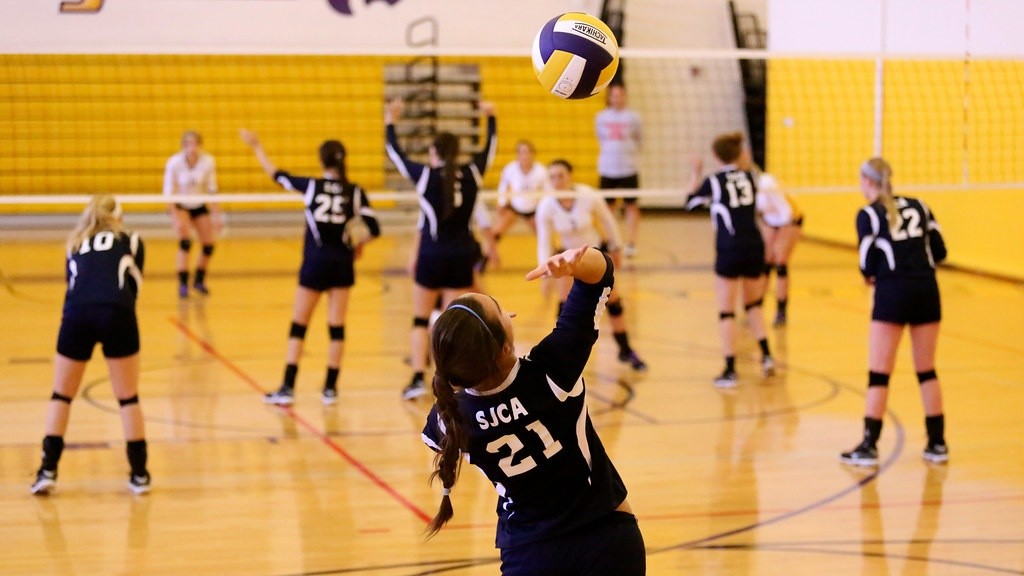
[531,12,620,100]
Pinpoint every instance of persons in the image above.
[476,140,548,272]
[386,94,496,400]
[596,83,643,255]
[403,186,500,367]
[420,244,647,576]
[164,131,217,295]
[29,195,153,494]
[682,132,776,385]
[745,160,801,327]
[241,128,381,405]
[840,157,950,464]
[534,158,647,368]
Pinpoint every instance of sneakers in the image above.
[620,350,645,370]
[773,314,786,329]
[923,444,949,463]
[841,450,877,465]
[127,472,151,494]
[322,388,337,404]
[263,389,293,405]
[32,469,56,495]
[713,375,738,388]
[403,381,427,401]
[763,357,774,376]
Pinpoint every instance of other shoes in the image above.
[179,283,187,298]
[195,283,208,293]
